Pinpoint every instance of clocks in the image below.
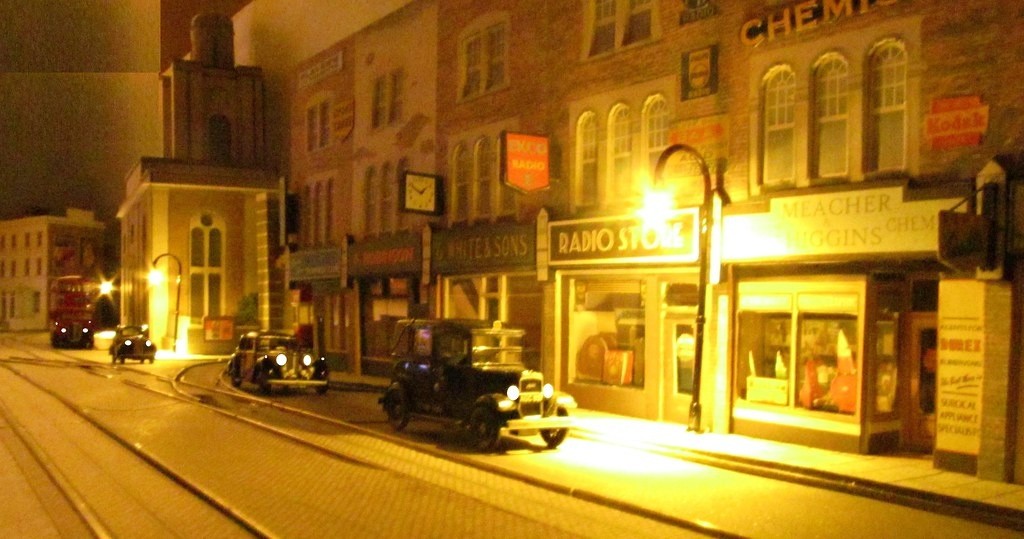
[398,169,445,218]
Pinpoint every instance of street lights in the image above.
[643,142,712,431]
[147,253,183,354]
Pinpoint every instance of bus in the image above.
[46,274,96,351]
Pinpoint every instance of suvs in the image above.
[228,329,330,399]
[377,317,578,453]
[108,323,157,365]
